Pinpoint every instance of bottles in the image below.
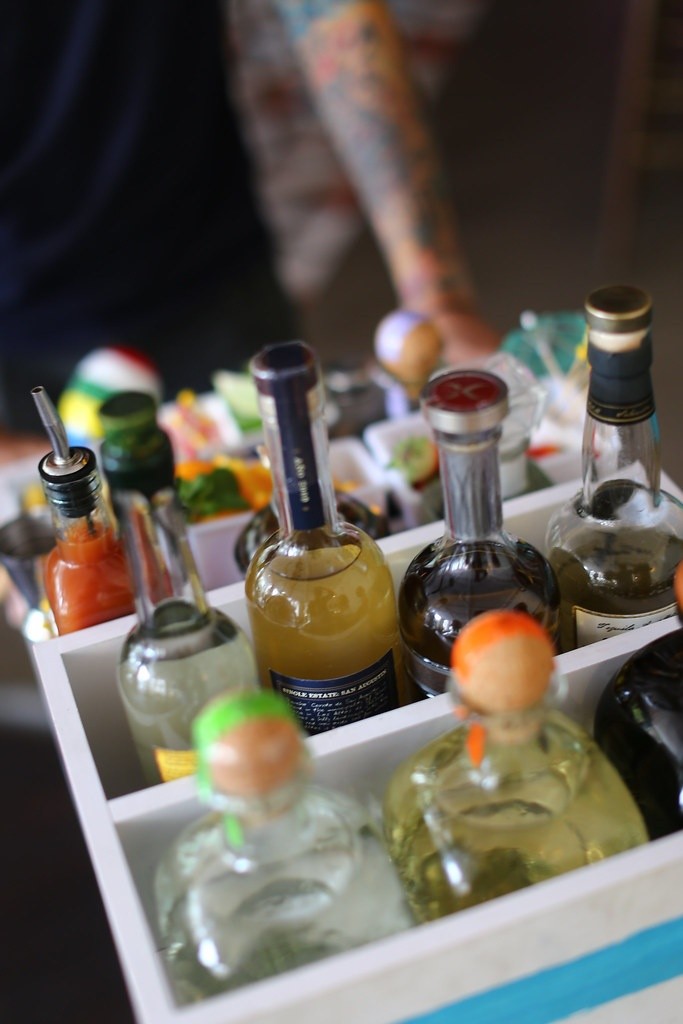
[0,283,683,1011]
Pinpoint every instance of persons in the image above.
[2,0,507,444]
